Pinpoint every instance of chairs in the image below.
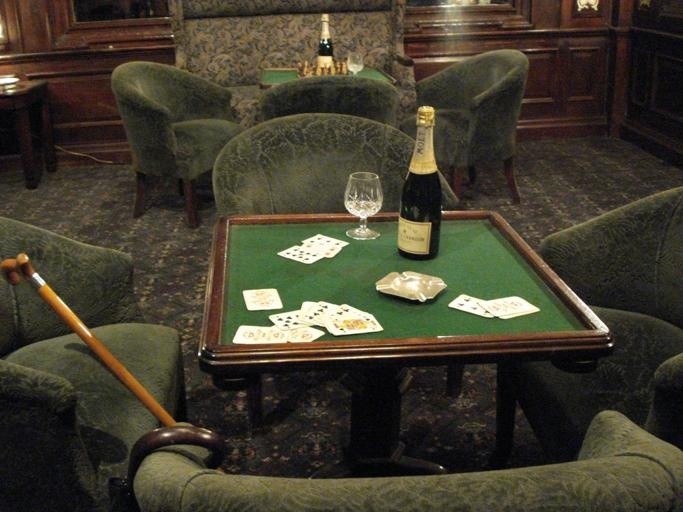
[132,408,682,512]
[212,113,463,420]
[494,183,683,454]
[0,214,187,512]
[416,49,531,208]
[241,73,404,130]
[109,60,244,222]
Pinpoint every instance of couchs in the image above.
[169,1,418,128]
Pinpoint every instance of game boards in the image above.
[296,60,348,79]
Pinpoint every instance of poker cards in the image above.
[231,288,384,345]
[448,293,541,319]
[276,233,350,263]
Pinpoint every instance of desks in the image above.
[258,66,399,91]
[197,208,613,480]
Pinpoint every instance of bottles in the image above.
[395,105,445,262]
[314,12,333,73]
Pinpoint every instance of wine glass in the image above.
[341,170,386,245]
[345,52,364,75]
